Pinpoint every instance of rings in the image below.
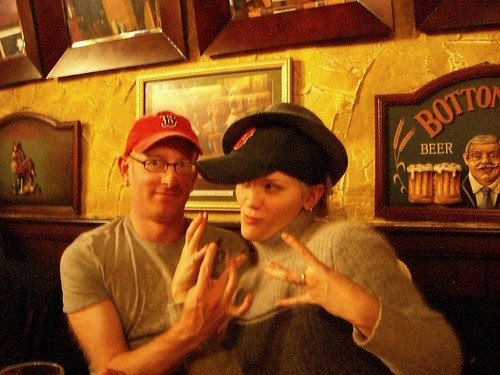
[300,272,307,286]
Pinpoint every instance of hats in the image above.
[124,110,204,159]
[221,103,349,188]
[200,123,331,187]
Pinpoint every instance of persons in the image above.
[57,110,259,375]
[459,134,500,209]
[169,102,465,375]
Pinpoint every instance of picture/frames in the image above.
[0,112,84,220]
[136,56,294,212]
[188,0,394,59]
[0,0,45,91]
[374,63,500,222]
[34,0,188,80]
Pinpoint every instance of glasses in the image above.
[129,155,195,175]
